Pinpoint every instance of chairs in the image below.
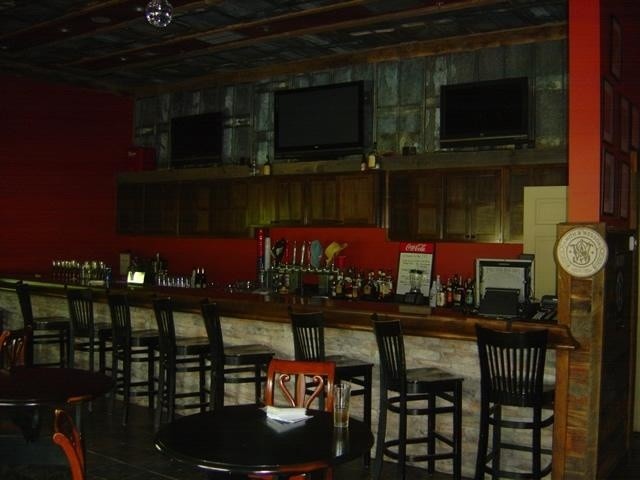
[64,282,114,417]
[199,296,276,411]
[474,322,560,479]
[15,279,72,367]
[368,312,465,479]
[105,287,160,427]
[152,299,212,433]
[2,324,33,366]
[264,356,336,414]
[52,408,88,479]
[286,303,375,469]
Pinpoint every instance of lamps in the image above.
[143,0,176,27]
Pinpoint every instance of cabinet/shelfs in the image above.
[110,178,178,237]
[501,163,569,244]
[246,172,385,234]
[443,164,504,247]
[385,169,444,243]
[178,181,262,239]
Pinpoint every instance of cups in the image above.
[332,428,350,459]
[334,380,351,430]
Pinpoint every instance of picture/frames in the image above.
[472,256,536,309]
[393,250,435,301]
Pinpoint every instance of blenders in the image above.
[402,267,427,304]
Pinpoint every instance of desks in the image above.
[2,365,118,478]
[154,404,374,478]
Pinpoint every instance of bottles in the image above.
[155,265,208,291]
[47,257,113,282]
[429,272,476,308]
[328,266,394,299]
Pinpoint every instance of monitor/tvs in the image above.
[440,77,529,148]
[274,79,364,161]
[171,112,222,158]
